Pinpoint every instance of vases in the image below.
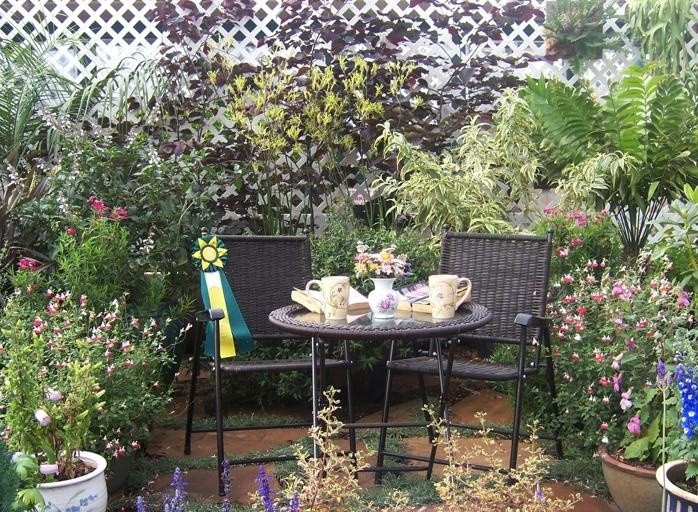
[31,448,134,511]
[597,446,697,511]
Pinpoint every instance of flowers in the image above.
[559,259,698,485]
[0,287,192,478]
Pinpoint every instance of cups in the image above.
[306,276,350,320]
[428,274,472,319]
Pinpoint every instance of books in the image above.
[392,280,430,312]
[289,284,370,314]
[411,296,432,313]
[392,309,411,319]
[410,311,444,324]
[293,310,367,326]
